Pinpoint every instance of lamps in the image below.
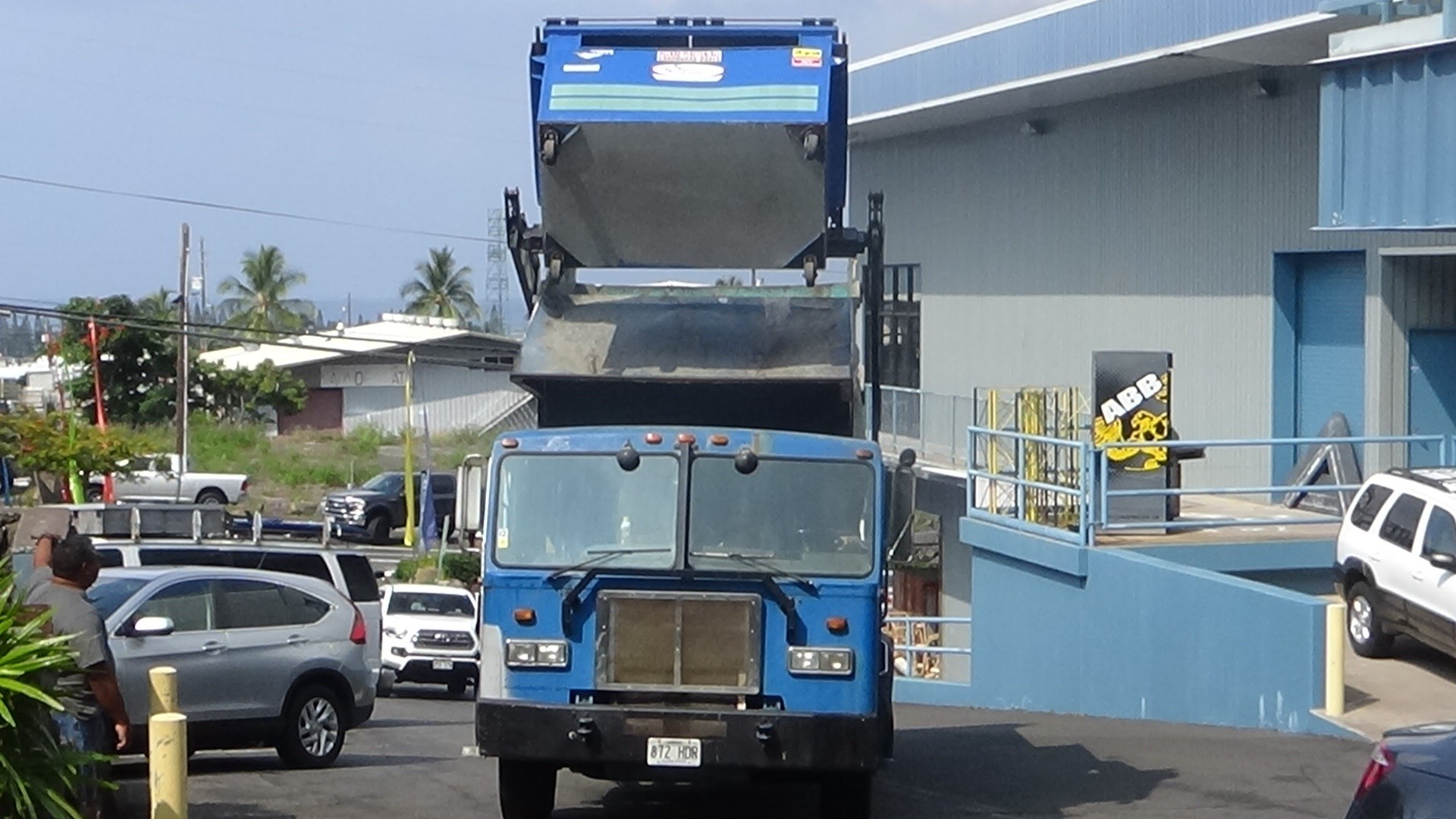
[1255,81,1286,98]
[1021,122,1046,136]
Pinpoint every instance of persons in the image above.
[25,532,131,819]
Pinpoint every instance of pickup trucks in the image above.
[83,452,249,506]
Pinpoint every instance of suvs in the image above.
[10,539,384,679]
[377,584,484,698]
[1330,463,1456,661]
[321,472,456,544]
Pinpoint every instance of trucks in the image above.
[452,8,918,819]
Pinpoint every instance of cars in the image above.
[1344,721,1456,819]
[87,568,376,769]
